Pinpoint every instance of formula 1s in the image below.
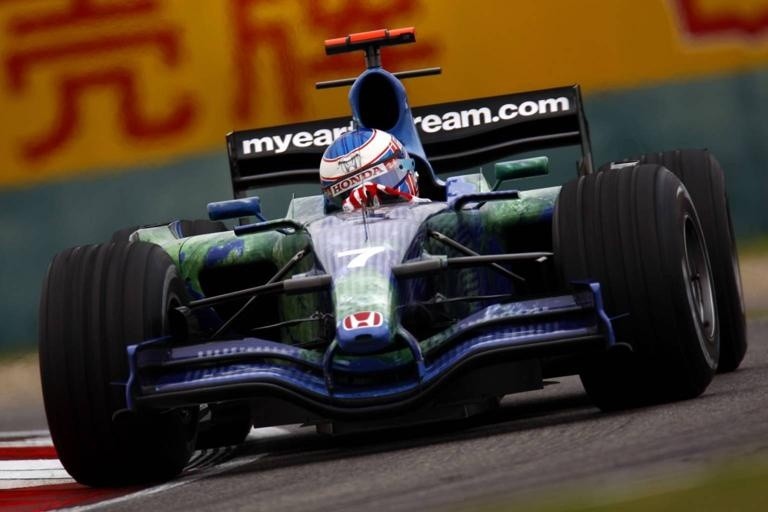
[36,16,748,484]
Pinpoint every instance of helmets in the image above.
[319,129,421,214]
